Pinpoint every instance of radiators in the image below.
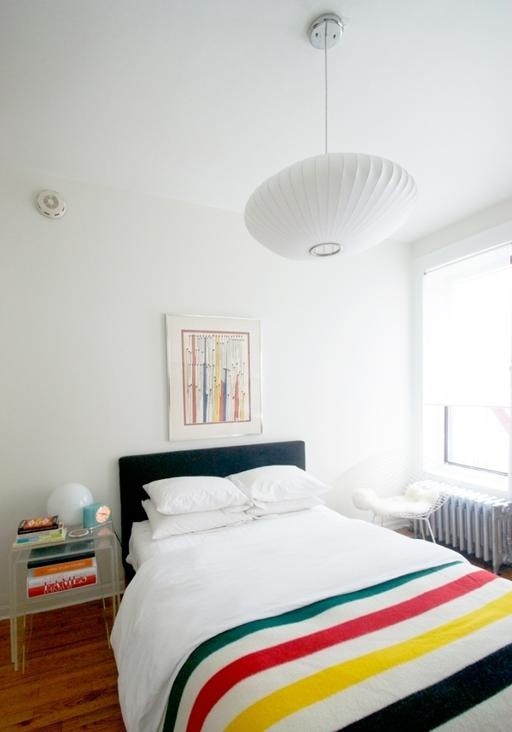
[412,489,512,573]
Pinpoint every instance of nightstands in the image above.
[0,526,126,673]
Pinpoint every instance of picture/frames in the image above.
[164,312,263,442]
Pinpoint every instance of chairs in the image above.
[349,482,452,543]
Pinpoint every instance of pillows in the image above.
[142,497,247,538]
[226,464,322,505]
[248,498,317,515]
[143,475,252,515]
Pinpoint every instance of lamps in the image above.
[243,13,420,264]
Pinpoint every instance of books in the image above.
[12,516,65,548]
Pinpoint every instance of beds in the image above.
[110,439,512,731]
